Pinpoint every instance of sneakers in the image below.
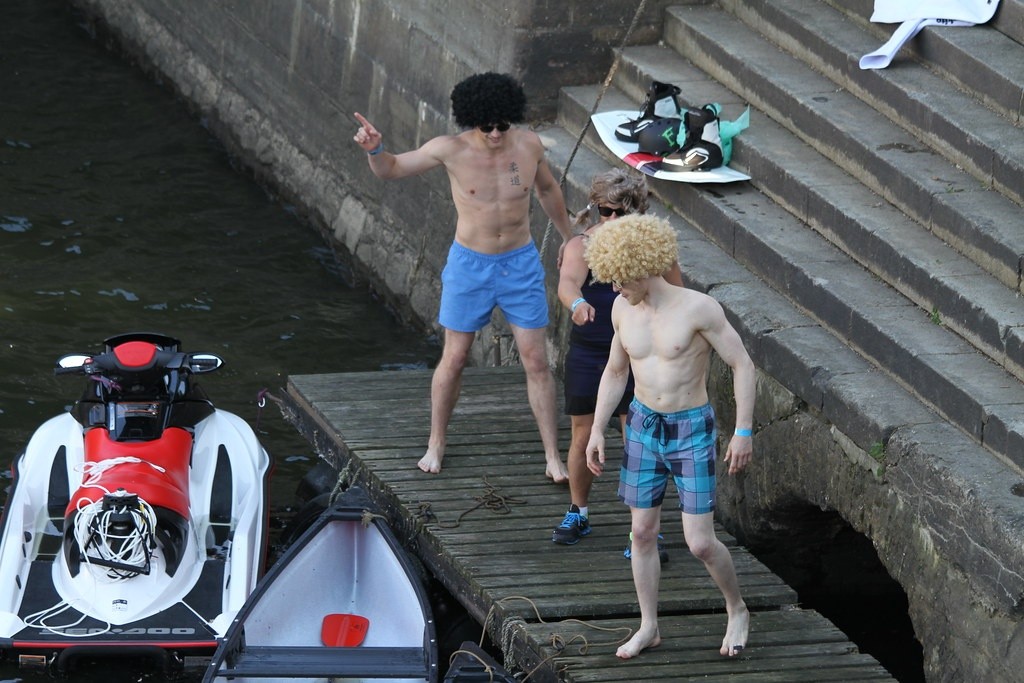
[553,504,591,545]
[623,532,665,558]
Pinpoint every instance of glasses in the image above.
[597,203,625,216]
[479,123,512,133]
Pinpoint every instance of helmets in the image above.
[638,117,681,155]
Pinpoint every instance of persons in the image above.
[558,168,683,565]
[353,73,573,483]
[584,213,755,659]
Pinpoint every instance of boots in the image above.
[663,104,723,172]
[616,81,682,142]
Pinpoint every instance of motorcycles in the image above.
[2,330,270,682]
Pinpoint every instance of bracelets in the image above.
[735,428,751,437]
[571,298,586,312]
[370,144,382,155]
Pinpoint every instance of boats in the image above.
[200,484,442,683]
